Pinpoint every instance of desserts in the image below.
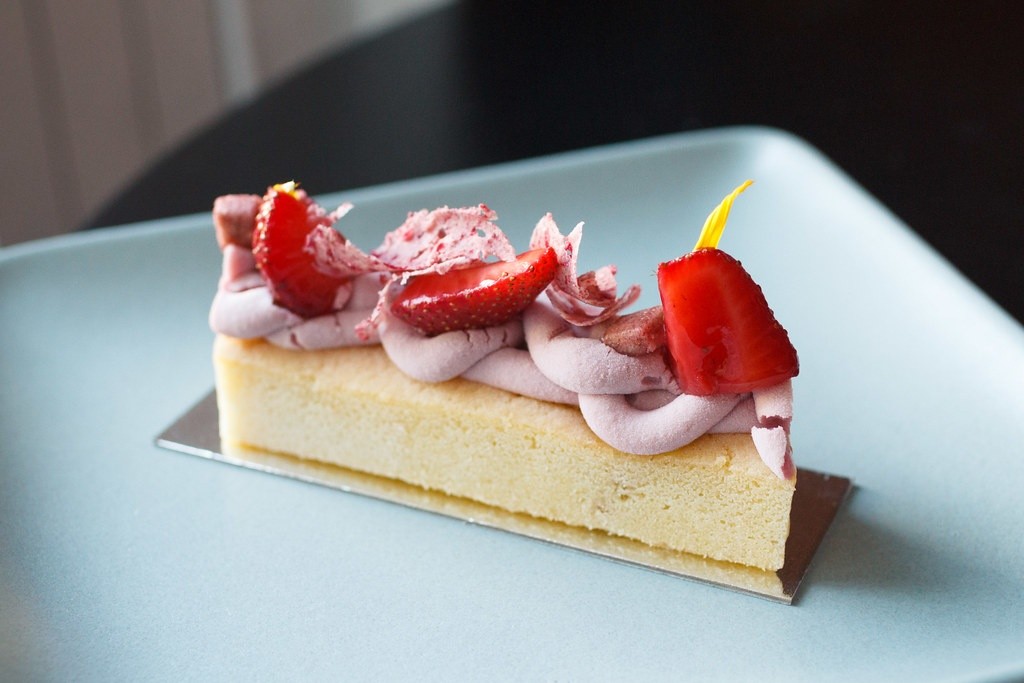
[205,180,801,573]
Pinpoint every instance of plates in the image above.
[0,125,1024,683]
